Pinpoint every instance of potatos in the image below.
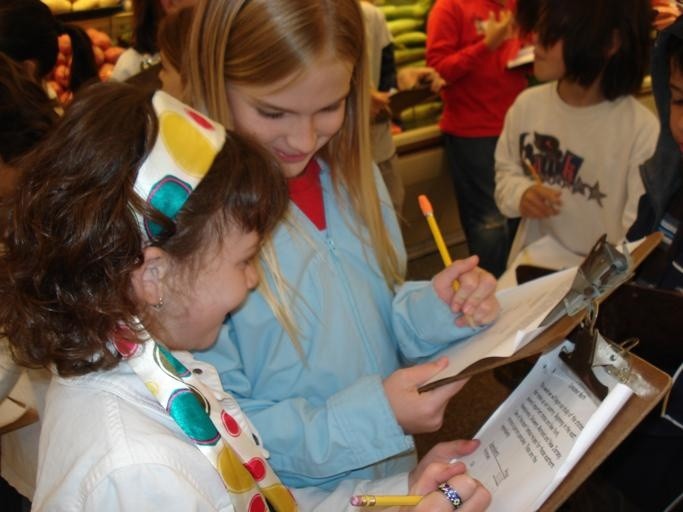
[45,27,127,106]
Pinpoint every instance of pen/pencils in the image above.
[350,495,424,507]
[524,157,550,207]
[369,81,392,116]
[418,194,478,333]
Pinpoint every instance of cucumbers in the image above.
[371,0,441,129]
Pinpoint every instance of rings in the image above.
[440,482,462,510]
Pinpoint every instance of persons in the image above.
[599,14,682,510]
[421,6,536,277]
[178,3,504,511]
[488,3,661,286]
[0,77,491,512]
